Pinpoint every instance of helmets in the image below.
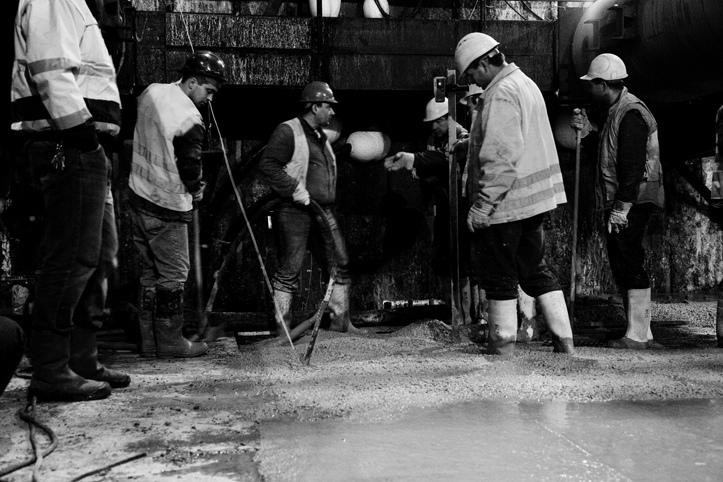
[186,50,226,82]
[455,32,500,105]
[300,80,339,105]
[423,96,449,122]
[579,52,629,82]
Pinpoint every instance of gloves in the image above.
[569,108,593,139]
[607,200,633,234]
[467,200,497,233]
[384,151,415,173]
[293,183,311,206]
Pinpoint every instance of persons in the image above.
[571,52,665,350]
[8,1,132,404]
[255,81,372,337]
[123,50,227,359]
[0,316,26,399]
[383,31,573,355]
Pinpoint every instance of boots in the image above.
[517,284,540,343]
[328,284,368,334]
[26,321,131,403]
[538,290,575,354]
[273,290,294,335]
[137,287,209,359]
[608,287,654,349]
[487,298,518,354]
